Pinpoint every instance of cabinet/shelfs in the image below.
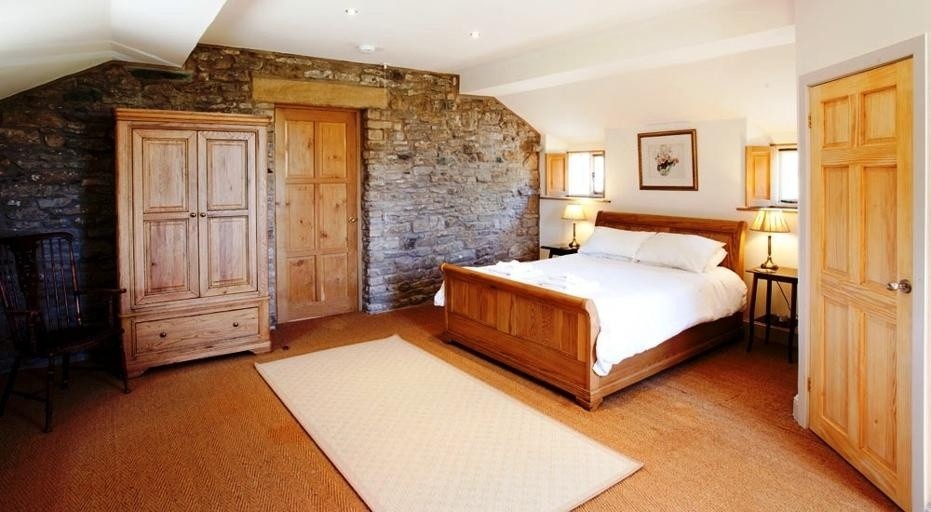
[112,109,274,379]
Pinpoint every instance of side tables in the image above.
[745,268,798,364]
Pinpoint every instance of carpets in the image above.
[254,334,644,512]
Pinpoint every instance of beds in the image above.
[439,210,747,411]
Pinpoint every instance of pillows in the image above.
[638,248,728,273]
[635,232,727,274]
[577,226,657,258]
[578,254,632,262]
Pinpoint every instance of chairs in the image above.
[0,231,132,434]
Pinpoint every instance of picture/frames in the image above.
[637,129,698,191]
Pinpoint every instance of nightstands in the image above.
[541,243,580,259]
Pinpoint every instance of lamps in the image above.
[561,204,588,248]
[749,207,790,269]
